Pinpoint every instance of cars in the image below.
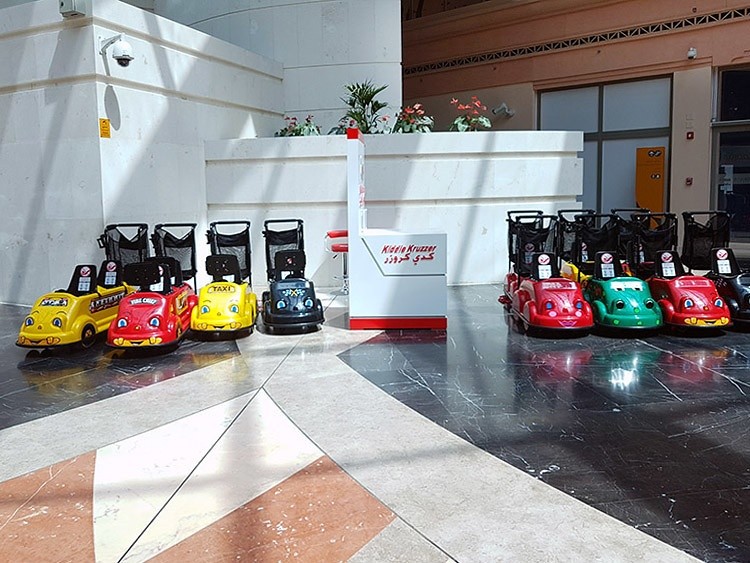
[189,220,262,337]
[16,224,198,352]
[261,219,324,334]
[500,208,750,333]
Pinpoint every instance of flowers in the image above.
[272,113,322,136]
[449,95,491,131]
[392,102,435,133]
[325,77,393,135]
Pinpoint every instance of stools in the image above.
[324,230,349,295]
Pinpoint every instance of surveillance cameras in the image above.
[113,41,134,67]
[687,50,695,59]
